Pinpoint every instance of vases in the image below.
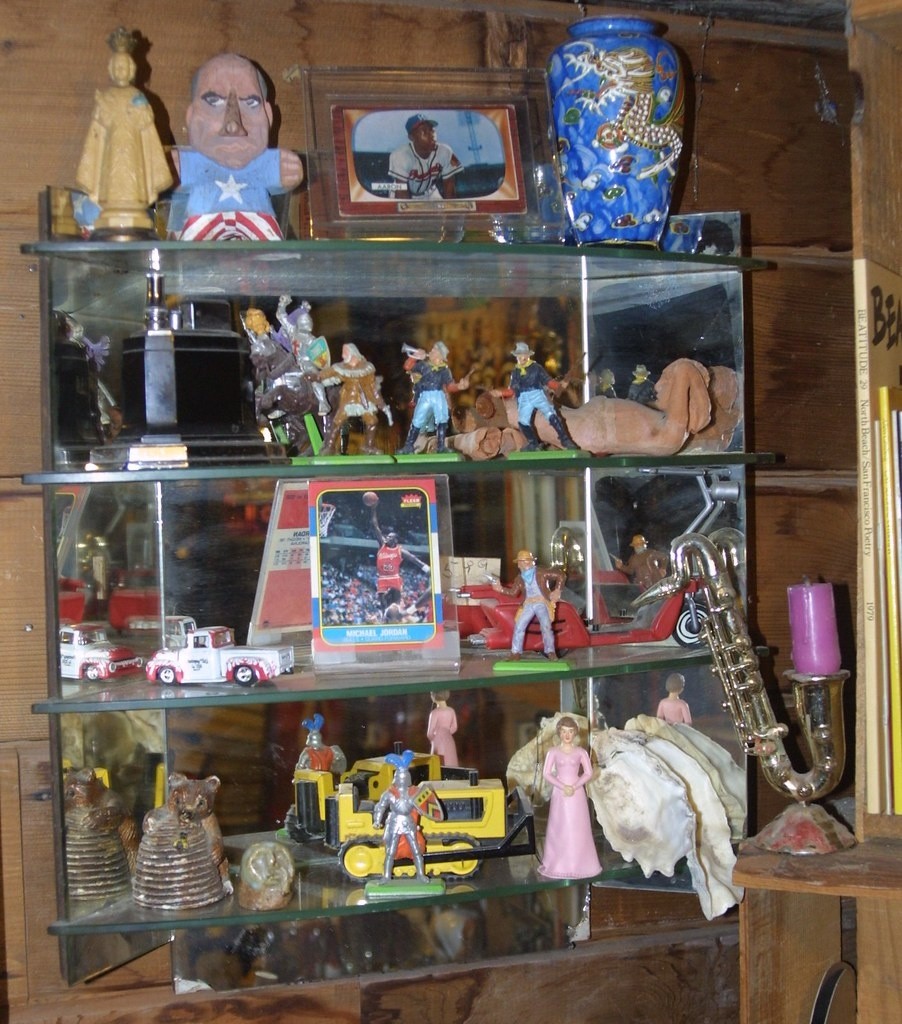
[549,19,681,250]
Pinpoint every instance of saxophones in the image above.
[629,535,853,805]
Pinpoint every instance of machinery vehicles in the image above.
[280,738,538,885]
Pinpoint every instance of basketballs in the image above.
[362,492,377,506]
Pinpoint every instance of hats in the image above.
[405,114,438,132]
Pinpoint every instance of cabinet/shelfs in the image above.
[491,0,902,1024]
[21,185,746,995]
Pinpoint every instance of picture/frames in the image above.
[299,65,565,246]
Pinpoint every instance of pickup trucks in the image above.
[144,623,298,689]
[57,622,146,684]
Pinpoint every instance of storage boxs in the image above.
[657,211,742,253]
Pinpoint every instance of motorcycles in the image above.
[443,525,714,658]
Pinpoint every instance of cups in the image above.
[492,162,565,244]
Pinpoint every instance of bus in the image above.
[124,612,198,650]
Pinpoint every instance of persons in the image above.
[309,341,393,456]
[595,364,660,403]
[167,52,304,240]
[426,687,461,768]
[397,341,460,457]
[537,715,605,880]
[658,674,697,726]
[296,715,348,775]
[610,537,671,596]
[243,294,332,452]
[511,342,582,453]
[389,114,468,200]
[485,549,566,663]
[324,492,432,625]
[373,749,433,887]
[76,27,173,243]
